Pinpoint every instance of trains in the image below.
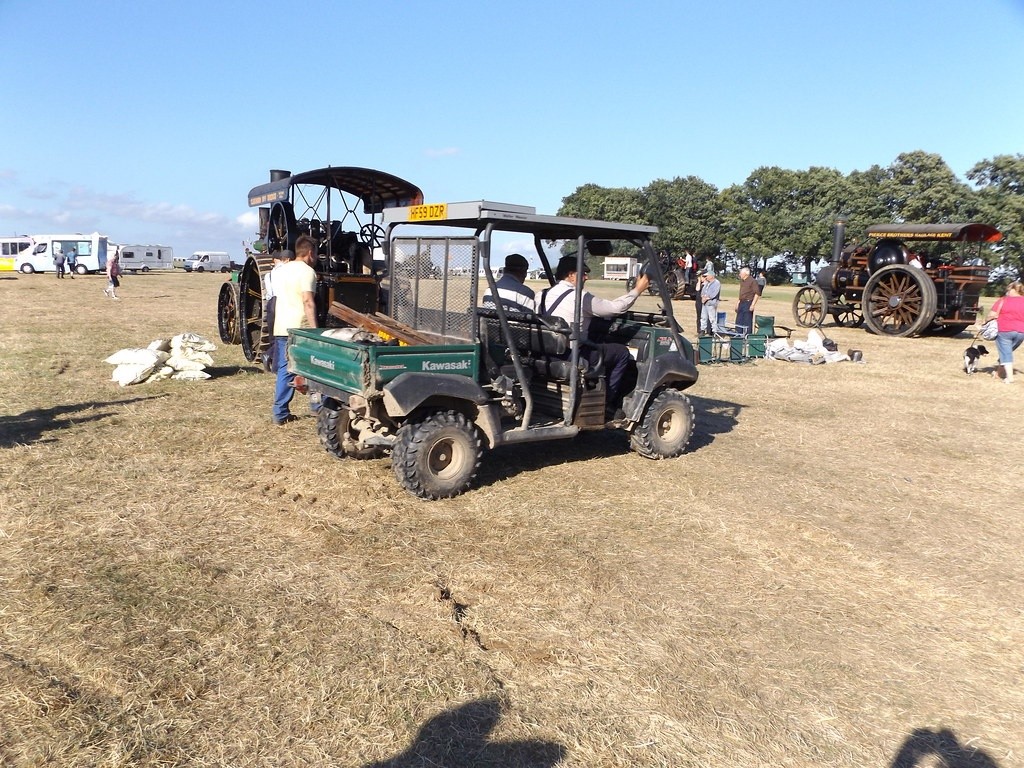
[791,218,1003,340]
[216,165,426,371]
[625,245,701,301]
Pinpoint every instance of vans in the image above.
[14,231,108,276]
[183,251,231,273]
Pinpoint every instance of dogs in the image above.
[963,345,989,373]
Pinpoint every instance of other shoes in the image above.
[104,290,109,297]
[605,407,626,419]
[992,371,1000,378]
[275,415,298,426]
[1004,378,1014,383]
[112,296,119,299]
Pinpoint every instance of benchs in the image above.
[467,307,606,391]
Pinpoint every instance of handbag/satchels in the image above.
[982,319,998,340]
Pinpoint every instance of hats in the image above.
[704,271,715,275]
[557,257,590,273]
[697,270,707,273]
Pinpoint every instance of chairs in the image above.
[697,312,796,366]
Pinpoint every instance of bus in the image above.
[0,236,34,274]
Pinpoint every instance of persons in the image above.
[56,250,66,279]
[682,250,692,283]
[695,271,721,335]
[264,249,295,374]
[756,271,766,298]
[272,236,327,426]
[535,257,648,419]
[103,255,123,299]
[735,267,760,334]
[483,253,535,324]
[982,281,1024,384]
[704,256,714,271]
[67,248,77,279]
